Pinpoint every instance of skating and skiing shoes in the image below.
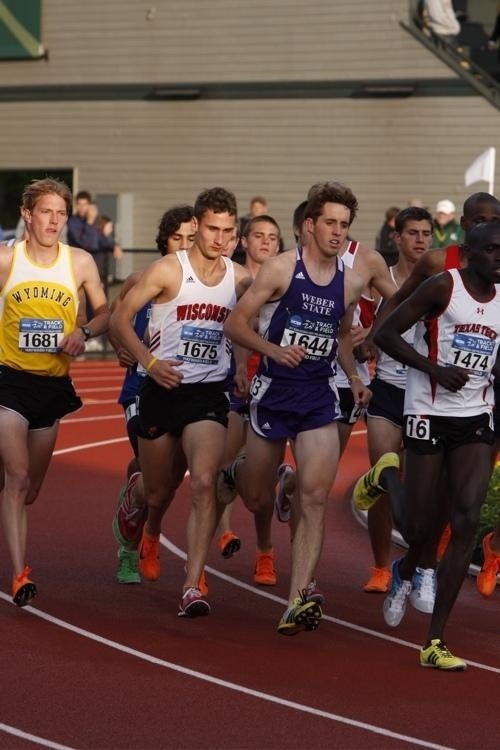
[364,566,392,594]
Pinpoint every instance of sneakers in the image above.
[113,471,148,549]
[409,566,438,616]
[420,637,466,673]
[137,445,299,619]
[353,452,400,511]
[277,588,323,636]
[477,532,499,598]
[11,563,38,607]
[117,546,142,585]
[307,579,325,604]
[382,556,413,629]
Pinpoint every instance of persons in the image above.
[416,0,500,76]
[0,177,109,608]
[108,181,499,674]
[66,190,121,351]
